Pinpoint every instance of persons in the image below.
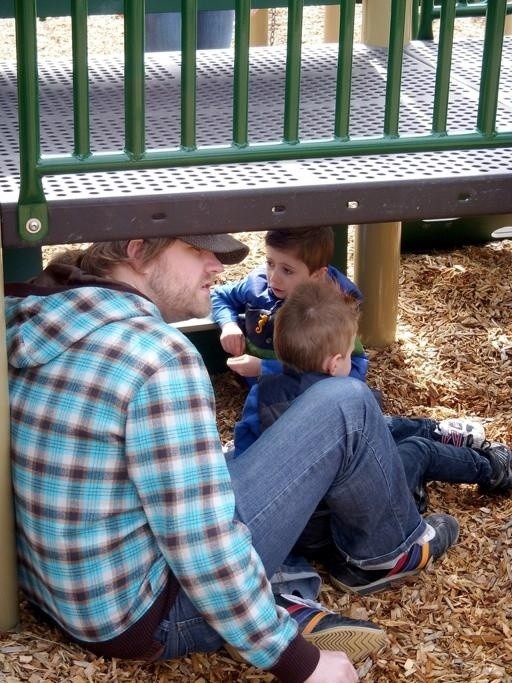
[5,233,460,683]
[234,280,512,514]
[210,225,368,382]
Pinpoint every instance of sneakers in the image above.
[480,441,511,498]
[428,412,490,450]
[268,592,385,664]
[332,513,464,595]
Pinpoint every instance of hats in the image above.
[171,232,251,268]
